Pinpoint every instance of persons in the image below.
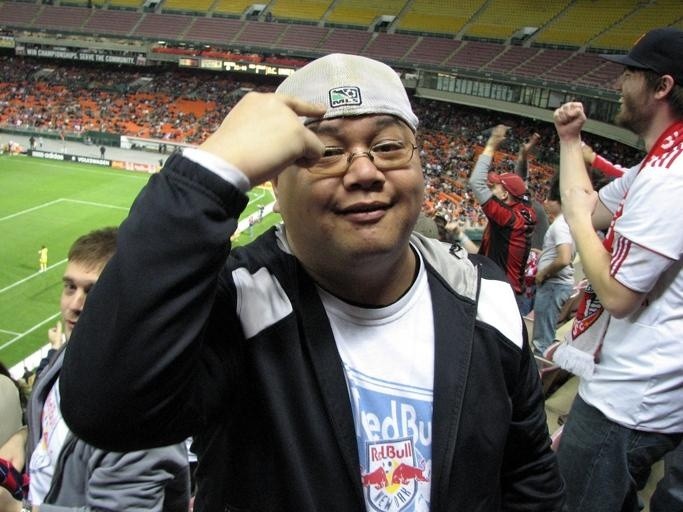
[0,227,198,512]
[552,27,682,512]
[0,28,649,359]
[59,52,567,512]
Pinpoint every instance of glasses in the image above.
[299,137,423,175]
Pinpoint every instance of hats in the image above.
[598,26,682,81]
[274,51,421,139]
[487,171,527,199]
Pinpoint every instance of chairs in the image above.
[531,273,599,463]
[0,3,683,229]
